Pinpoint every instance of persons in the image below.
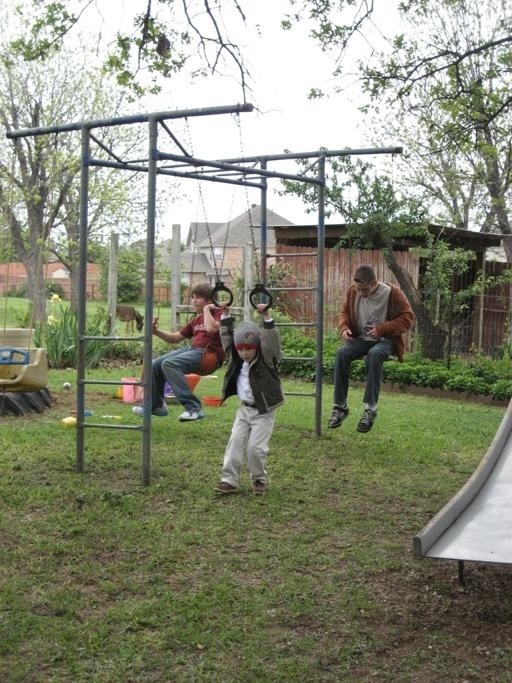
[328,265,415,432]
[213,303,285,496]
[132,283,224,421]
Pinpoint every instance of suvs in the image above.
[123,378,143,403]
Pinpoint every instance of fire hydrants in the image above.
[186,177,236,375]
[348,153,395,360]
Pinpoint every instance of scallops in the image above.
[116,305,144,333]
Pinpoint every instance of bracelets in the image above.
[201,396,224,407]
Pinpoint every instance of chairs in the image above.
[251,479,265,495]
[178,408,204,421]
[131,400,169,416]
[355,407,377,433]
[327,402,349,428]
[214,481,237,492]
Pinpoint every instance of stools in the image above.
[241,400,255,407]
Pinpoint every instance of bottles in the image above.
[232,320,261,349]
